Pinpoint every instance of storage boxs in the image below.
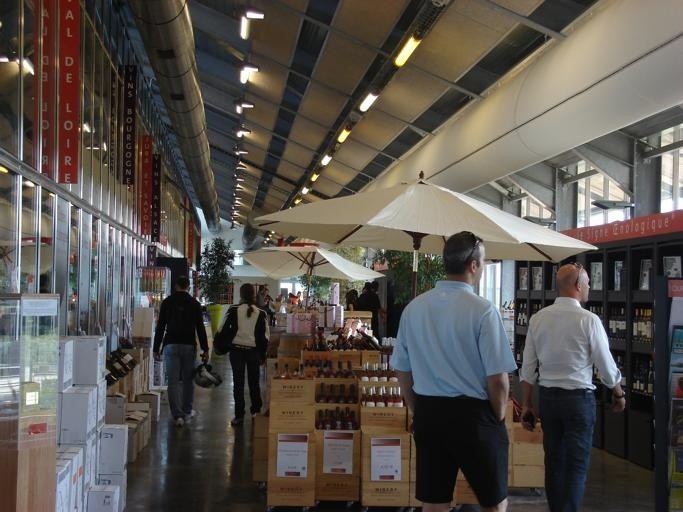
[53,334,161,511]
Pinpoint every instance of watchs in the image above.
[611,390,625,399]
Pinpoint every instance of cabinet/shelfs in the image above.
[514,208,683,470]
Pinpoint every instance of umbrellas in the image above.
[252,169,598,300]
[237,244,387,312]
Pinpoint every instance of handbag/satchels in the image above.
[212,308,236,356]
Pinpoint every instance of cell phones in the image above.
[523,411,535,428]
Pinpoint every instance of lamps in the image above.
[0,38,36,75]
[86,139,108,152]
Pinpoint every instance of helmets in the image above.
[195,364,222,387]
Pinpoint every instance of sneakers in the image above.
[231,416,244,426]
[176,417,184,428]
[184,414,192,424]
[250,407,257,418]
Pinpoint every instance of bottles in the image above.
[613,354,628,388]
[381,336,395,347]
[630,308,653,345]
[592,362,600,381]
[315,406,359,431]
[271,360,398,382]
[606,306,626,339]
[589,305,603,327]
[105,347,138,389]
[532,303,542,315]
[300,328,380,351]
[630,356,652,394]
[515,336,524,362]
[317,383,403,408]
[515,302,527,326]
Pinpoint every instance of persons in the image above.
[38,272,51,296]
[390,229,518,512]
[252,279,389,345]
[212,282,269,426]
[513,260,628,511]
[150,274,209,428]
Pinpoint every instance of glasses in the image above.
[572,261,583,283]
[463,228,481,264]
